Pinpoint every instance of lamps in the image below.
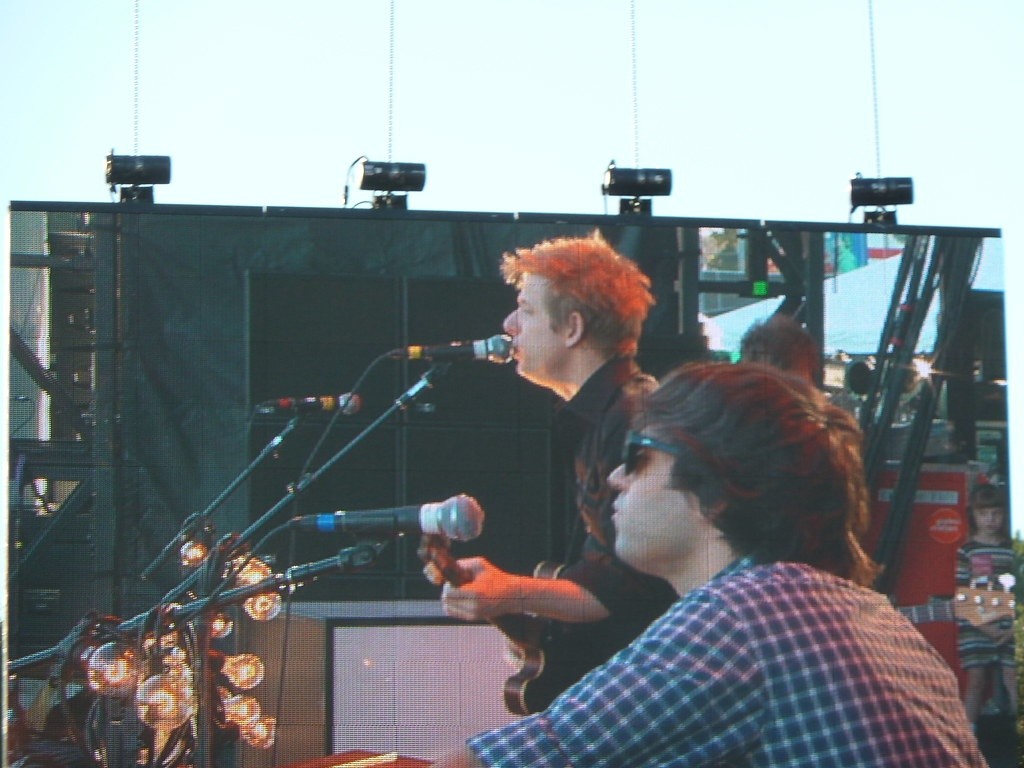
[104,151,171,203]
[601,166,672,216]
[353,161,426,210]
[848,175,914,225]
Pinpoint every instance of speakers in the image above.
[947,288,1007,423]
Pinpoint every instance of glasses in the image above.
[623,429,725,476]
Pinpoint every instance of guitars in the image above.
[416,529,601,719]
[896,570,1018,629]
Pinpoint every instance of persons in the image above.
[431,363,986,768]
[440,237,680,718]
[953,482,1020,728]
[738,321,816,389]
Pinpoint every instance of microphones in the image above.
[261,393,361,418]
[293,492,487,538]
[391,333,519,368]
